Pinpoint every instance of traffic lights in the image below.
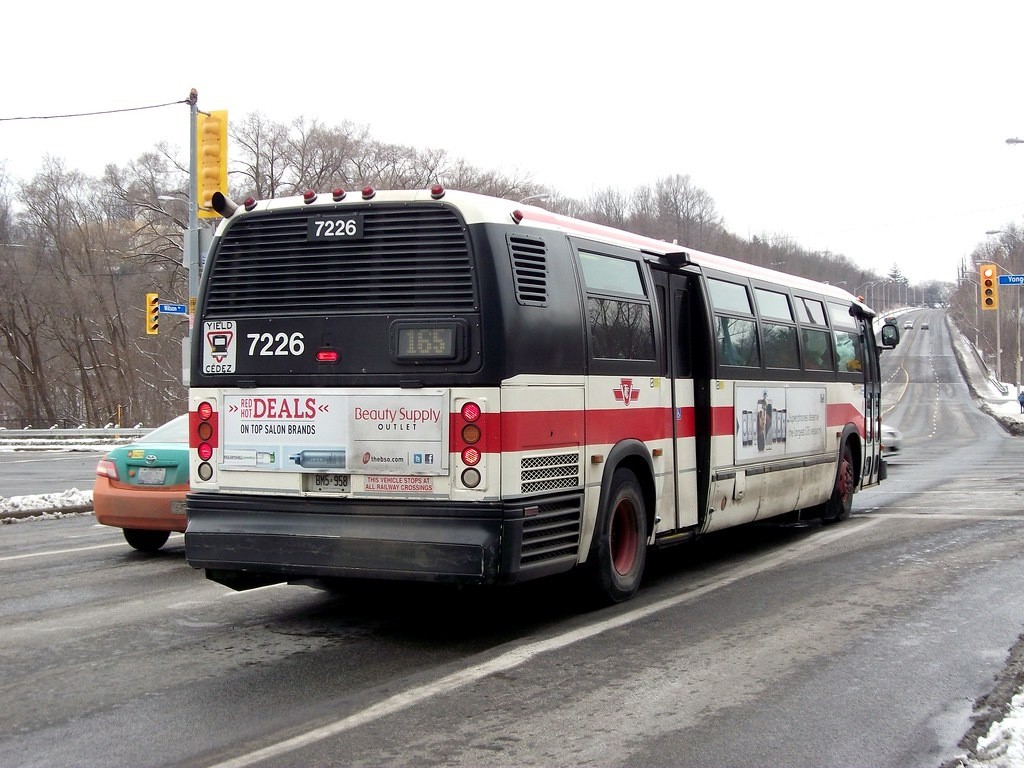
[146,293,159,335]
[980,265,999,311]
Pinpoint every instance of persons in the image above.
[757,403,772,451]
[1018,391,1024,414]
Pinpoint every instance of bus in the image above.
[184,184,904,606]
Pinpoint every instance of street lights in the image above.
[958,270,1002,382]
[975,259,1022,400]
[833,281,847,286]
[853,280,912,316]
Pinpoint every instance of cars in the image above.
[92,412,191,553]
[904,321,914,330]
[921,323,929,330]
[886,317,897,325]
